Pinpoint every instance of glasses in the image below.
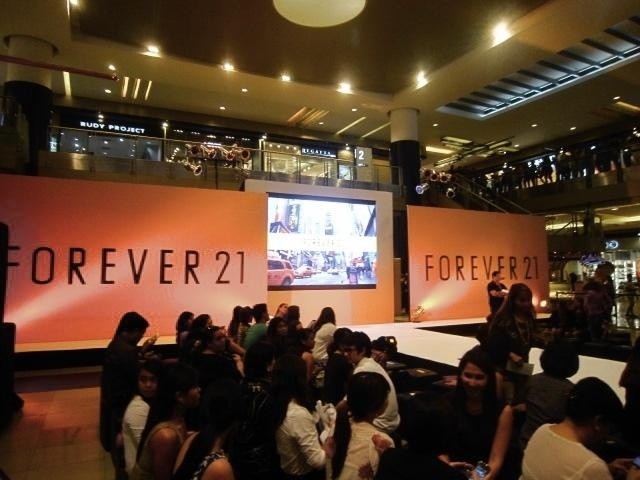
[345,348,356,352]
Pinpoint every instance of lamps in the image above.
[273,0,366,28]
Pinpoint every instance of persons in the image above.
[449,147,633,196]
[99,261,640,480]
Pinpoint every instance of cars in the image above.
[295,266,314,279]
[267,259,295,288]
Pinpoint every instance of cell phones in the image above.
[628,456,640,472]
[474,459,487,476]
